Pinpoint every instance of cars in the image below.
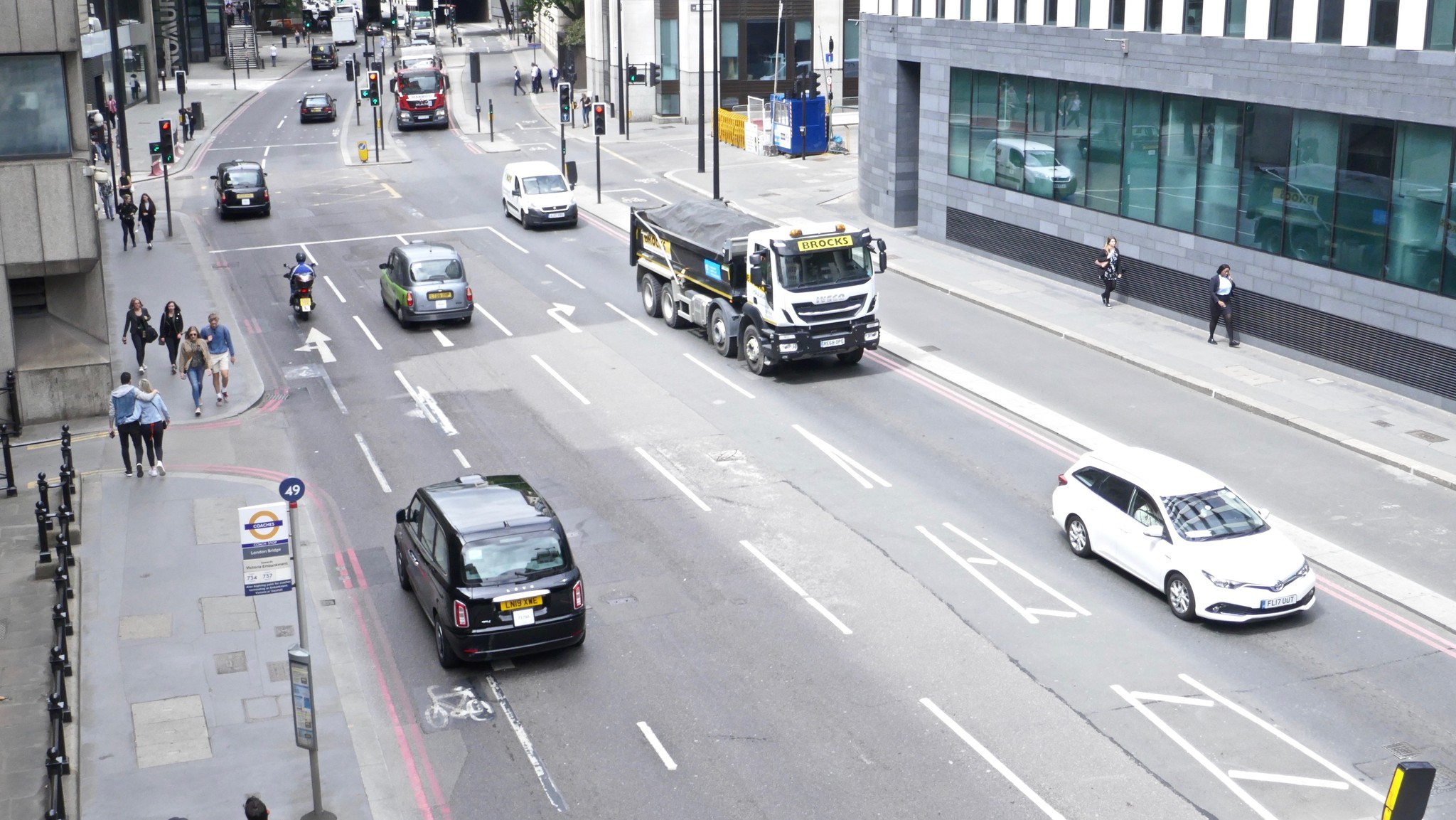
[378,240,474,329]
[394,474,587,672]
[210,159,270,221]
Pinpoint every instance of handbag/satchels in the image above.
[139,89,141,92]
[193,115,197,125]
[590,104,592,111]
[162,420,167,429]
[146,325,158,343]
[159,340,165,345]
[127,176,135,193]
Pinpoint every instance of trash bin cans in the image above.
[282,37,287,48]
[189,101,205,130]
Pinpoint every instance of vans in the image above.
[980,139,1078,201]
[502,161,578,230]
[760,61,812,81]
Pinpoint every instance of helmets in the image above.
[296,252,306,262]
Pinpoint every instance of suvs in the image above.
[1051,446,1317,623]
[1077,122,1159,168]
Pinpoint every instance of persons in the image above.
[109,372,160,478]
[138,193,156,250]
[184,108,197,141]
[89,122,120,167]
[179,326,213,415]
[529,62,544,95]
[269,43,277,67]
[508,22,513,40]
[116,194,137,251]
[118,170,134,204]
[99,177,115,221]
[226,0,250,27]
[295,30,301,47]
[513,65,526,96]
[521,18,533,37]
[160,300,184,373]
[549,65,561,92]
[283,252,316,310]
[244,797,270,820]
[1098,236,1122,308]
[453,24,459,43]
[200,313,235,406]
[106,95,118,128]
[575,93,591,128]
[130,74,141,99]
[123,378,170,476]
[317,19,328,34]
[123,298,152,373]
[301,27,309,47]
[1208,264,1240,347]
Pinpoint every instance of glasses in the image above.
[190,333,197,336]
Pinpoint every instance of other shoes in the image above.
[289,303,294,306]
[171,364,177,374]
[107,162,110,165]
[190,138,195,141]
[124,245,128,251]
[111,217,115,221]
[107,217,110,219]
[195,408,201,415]
[132,240,137,247]
[116,163,120,166]
[186,139,190,141]
[583,124,589,129]
[199,397,202,405]
[147,245,153,250]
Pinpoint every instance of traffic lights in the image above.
[561,140,566,151]
[808,71,821,100]
[149,119,174,164]
[175,70,186,94]
[306,16,310,29]
[391,13,396,25]
[451,10,455,21]
[649,62,660,87]
[629,66,645,82]
[490,104,493,114]
[361,70,382,107]
[592,102,607,136]
[558,82,572,124]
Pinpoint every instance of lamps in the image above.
[856,19,860,26]
[889,28,894,32]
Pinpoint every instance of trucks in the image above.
[265,0,456,131]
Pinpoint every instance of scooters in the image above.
[283,263,316,321]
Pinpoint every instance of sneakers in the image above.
[148,468,157,476]
[143,363,147,369]
[156,460,166,476]
[216,398,222,406]
[221,389,229,402]
[139,366,144,374]
[136,462,144,478]
[125,471,133,476]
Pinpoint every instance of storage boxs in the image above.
[295,273,314,289]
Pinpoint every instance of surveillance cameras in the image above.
[93,112,103,127]
[94,171,109,186]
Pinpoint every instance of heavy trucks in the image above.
[629,197,886,377]
[1245,162,1456,298]
